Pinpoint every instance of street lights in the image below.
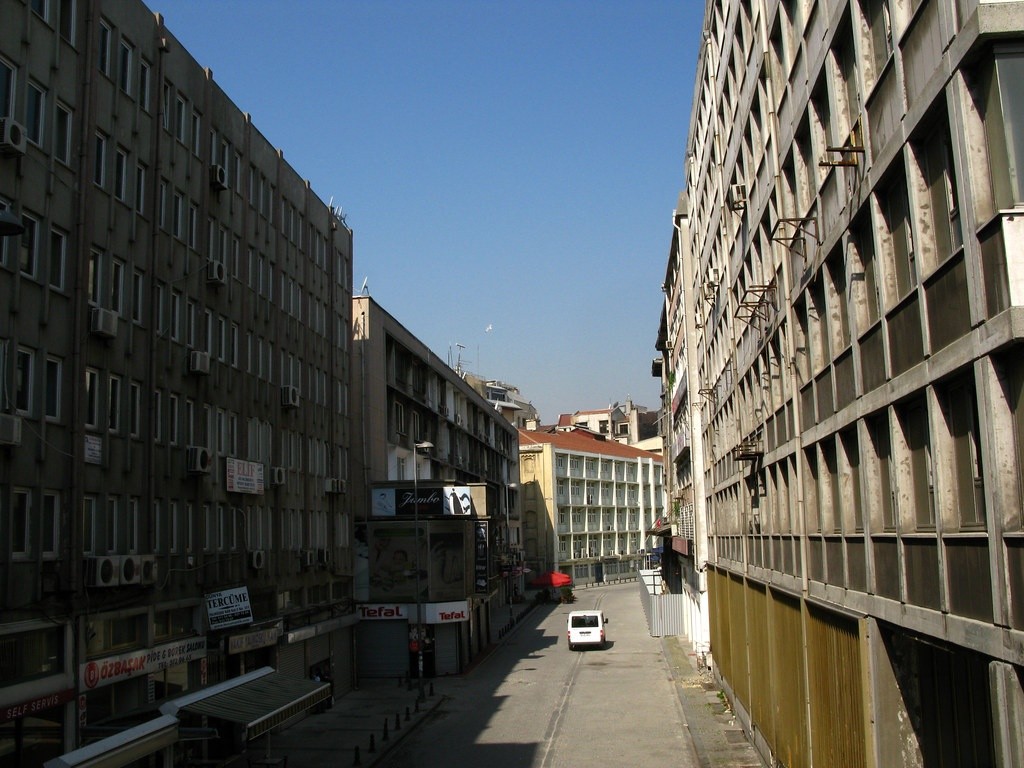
[411,442,433,701]
[504,482,517,628]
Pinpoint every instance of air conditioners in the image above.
[444,407,491,467]
[86,555,159,587]
[208,260,227,287]
[0,117,28,155]
[281,385,300,408]
[336,479,347,494]
[269,467,285,486]
[694,313,704,329]
[0,413,26,448]
[190,351,211,377]
[210,164,228,191]
[248,550,266,570]
[301,549,318,566]
[325,478,338,493]
[708,268,719,288]
[574,548,646,559]
[673,488,682,499]
[91,308,118,339]
[187,446,213,475]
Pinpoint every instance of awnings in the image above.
[175,672,332,742]
[645,525,671,538]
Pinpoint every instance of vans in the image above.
[566,610,608,650]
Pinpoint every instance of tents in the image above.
[543,586,550,605]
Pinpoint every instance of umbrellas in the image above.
[528,568,572,602]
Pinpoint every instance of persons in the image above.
[392,549,408,570]
[350,529,386,565]
